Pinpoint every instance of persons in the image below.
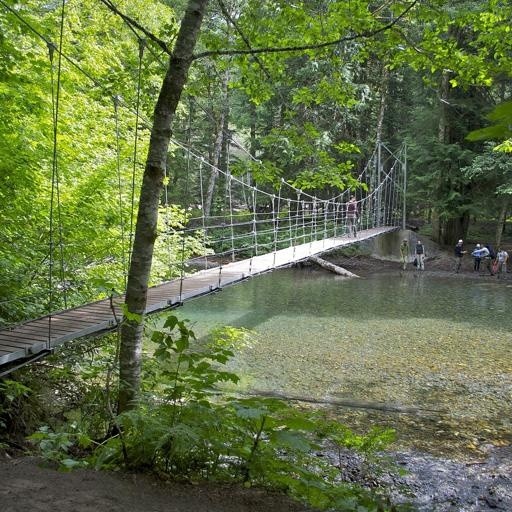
[345,195,360,238]
[495,246,509,279]
[455,239,468,273]
[415,240,425,271]
[471,244,483,271]
[399,239,409,270]
